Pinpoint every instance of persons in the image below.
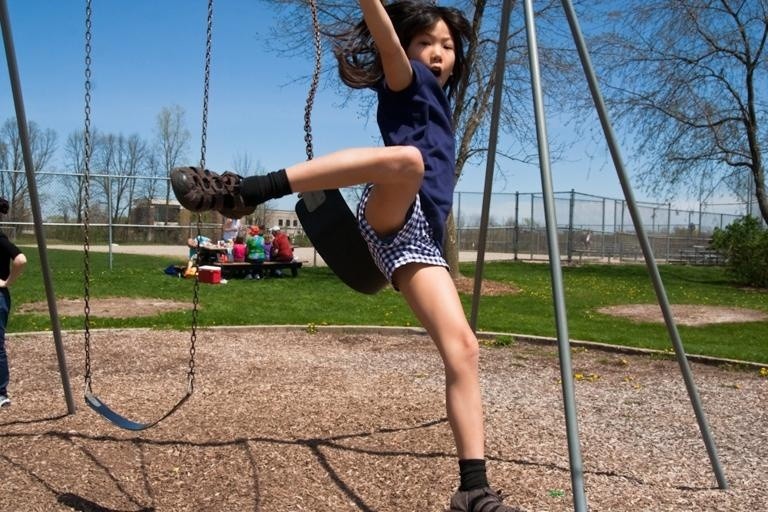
[244,225,266,280]
[267,227,295,278]
[167,3,526,511]
[262,232,273,260]
[1,194,27,406]
[231,235,248,277]
[186,234,212,268]
[222,214,240,245]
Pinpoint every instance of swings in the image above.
[297,1,480,294]
[84,0,210,429]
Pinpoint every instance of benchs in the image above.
[213,259,308,278]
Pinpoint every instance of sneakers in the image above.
[0,396,10,407]
[254,274,259,280]
[170,165,255,219]
[449,485,522,512]
[244,274,252,280]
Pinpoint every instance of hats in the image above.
[251,226,258,232]
[269,226,280,231]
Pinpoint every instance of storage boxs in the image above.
[198,265,221,283]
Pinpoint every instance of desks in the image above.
[201,245,299,277]
